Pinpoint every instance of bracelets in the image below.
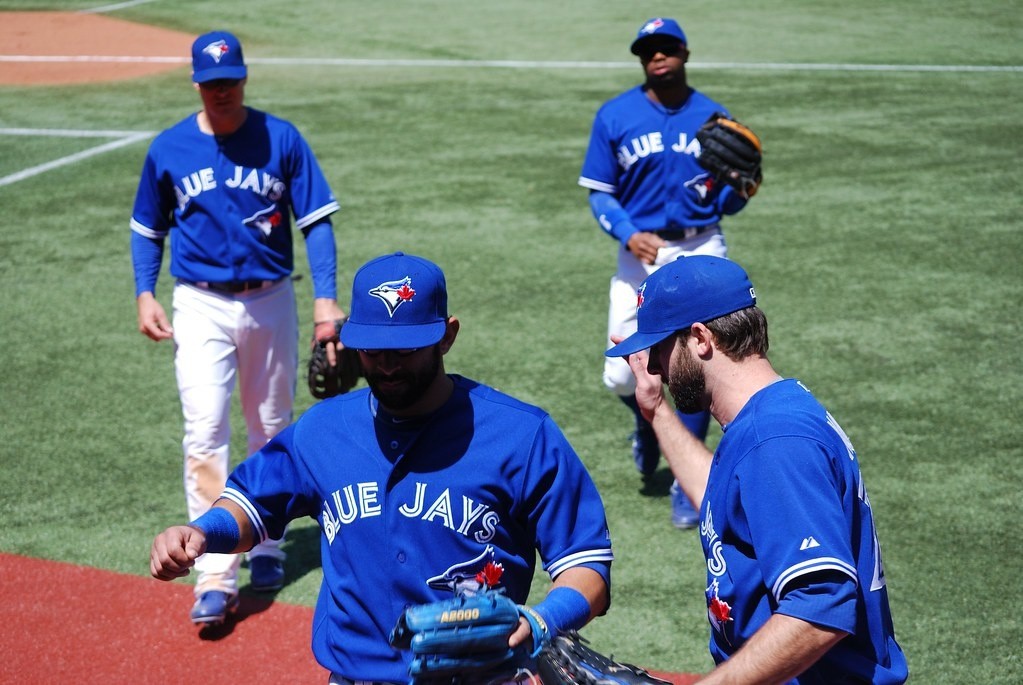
[188,509,241,556]
[539,586,590,636]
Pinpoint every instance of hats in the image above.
[632,19,687,55]
[340,253,448,350]
[605,254,759,357]
[192,31,248,84]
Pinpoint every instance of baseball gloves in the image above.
[391,586,681,684]
[697,110,764,200]
[307,320,357,397]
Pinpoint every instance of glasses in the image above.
[203,78,240,88]
[362,349,417,355]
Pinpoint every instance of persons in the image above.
[579,17,764,532]
[152,253,614,685]
[128,30,351,629]
[603,252,911,685]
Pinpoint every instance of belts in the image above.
[647,223,710,242]
[186,276,273,294]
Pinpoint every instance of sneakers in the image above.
[190,590,242,626]
[671,477,702,529]
[635,427,660,475]
[250,554,285,591]
